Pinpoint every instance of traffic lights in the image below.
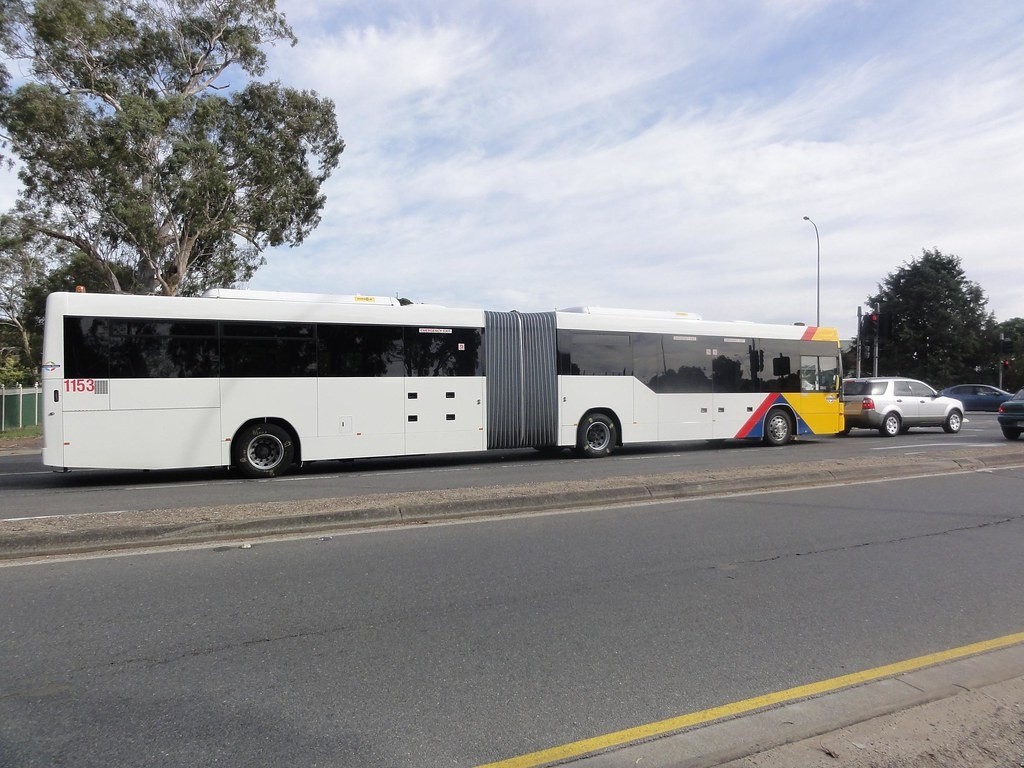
[1005,359,1012,365]
[871,313,878,336]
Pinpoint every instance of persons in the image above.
[977,387,988,395]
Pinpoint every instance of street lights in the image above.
[803,216,820,326]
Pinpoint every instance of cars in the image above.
[842,377,965,438]
[996,385,1024,440]
[939,383,1016,412]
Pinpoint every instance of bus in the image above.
[42,285,845,480]
